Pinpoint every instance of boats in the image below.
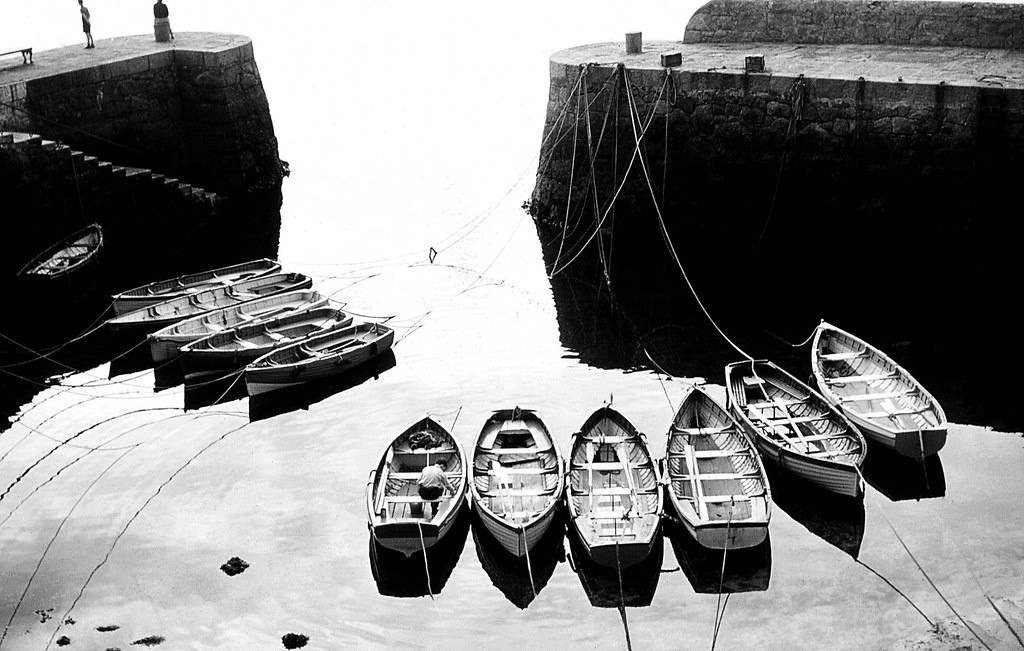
[14,220,108,288]
[468,406,565,559]
[364,412,468,558]
[245,319,395,397]
[145,288,333,365]
[565,402,668,571]
[177,304,356,391]
[103,272,315,333]
[661,382,775,549]
[810,317,950,461]
[724,357,869,501]
[110,256,284,316]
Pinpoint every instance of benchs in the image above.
[578,488,656,496]
[843,388,915,401]
[0,48,33,65]
[583,436,637,444]
[70,243,92,246]
[690,494,765,503]
[58,256,83,259]
[488,469,543,474]
[788,431,847,446]
[42,266,66,269]
[678,448,749,458]
[680,471,759,479]
[205,321,223,330]
[388,496,447,502]
[229,292,248,300]
[236,311,259,322]
[866,406,927,419]
[213,272,232,285]
[492,448,538,453]
[679,428,736,435]
[824,351,869,361]
[586,510,653,519]
[302,350,322,356]
[828,373,897,385]
[488,488,545,496]
[754,397,807,410]
[813,446,858,458]
[574,463,649,470]
[768,411,828,426]
[264,330,286,341]
[191,301,218,310]
[389,472,465,477]
[233,338,257,348]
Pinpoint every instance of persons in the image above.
[78,0,94,49]
[154,0,174,39]
[416,458,456,517]
